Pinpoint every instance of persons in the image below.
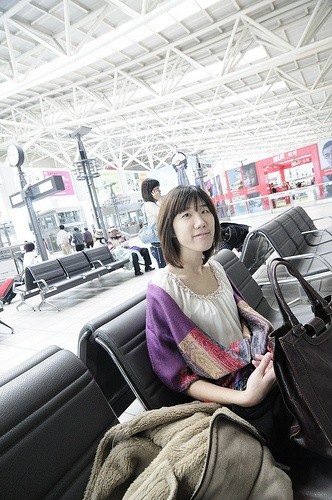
[321,140,332,196]
[20,178,177,281]
[146,184,291,439]
[242,164,260,199]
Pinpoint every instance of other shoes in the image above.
[135,271,143,275]
[145,266,155,272]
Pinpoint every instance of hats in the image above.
[94,229,104,238]
[108,228,118,233]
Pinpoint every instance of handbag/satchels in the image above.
[267,258,332,460]
[138,204,160,244]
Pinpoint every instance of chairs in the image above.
[0,247,332,500]
[237,204,332,305]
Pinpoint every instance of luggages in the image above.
[0,271,25,305]
